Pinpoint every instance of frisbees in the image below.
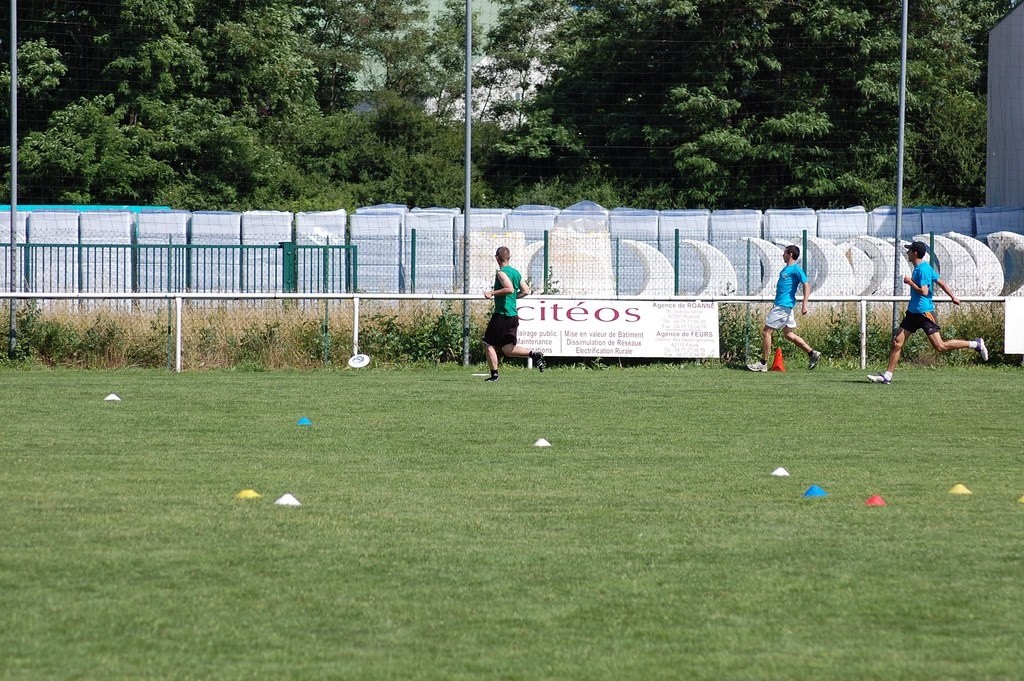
[348,353,370,368]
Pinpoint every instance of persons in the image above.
[868,242,989,385]
[747,245,821,373]
[483,246,545,381]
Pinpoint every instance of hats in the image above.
[904,241,927,259]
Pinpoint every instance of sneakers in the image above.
[974,338,989,362]
[867,372,892,385]
[747,361,768,373]
[806,351,822,371]
[534,352,546,373]
[483,375,500,383]
